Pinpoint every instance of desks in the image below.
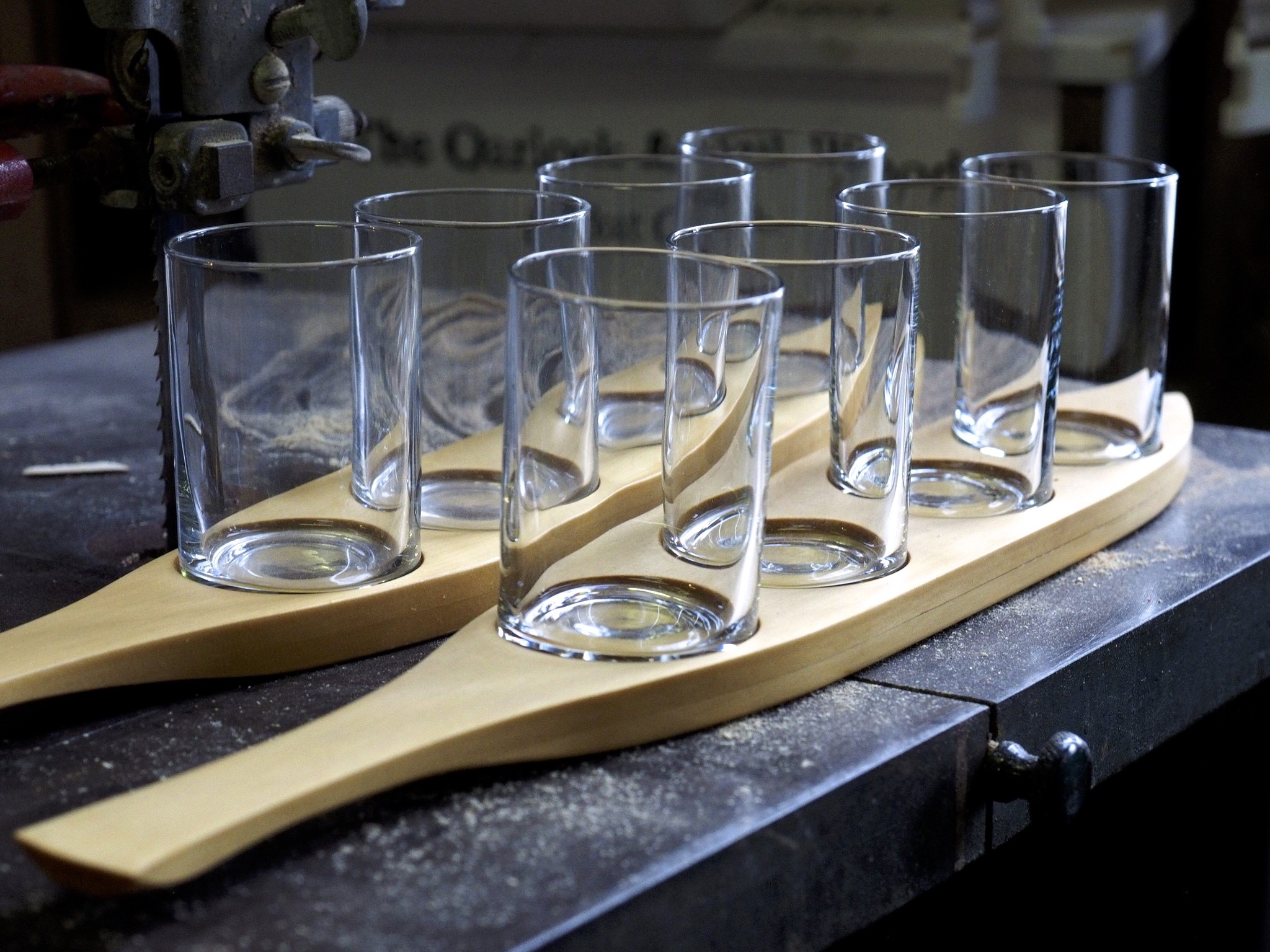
[3,282,1268,952]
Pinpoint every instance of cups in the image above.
[824,175,1071,518]
[680,124,887,398]
[535,153,754,431]
[660,215,921,586]
[350,189,602,530]
[491,248,788,666]
[162,222,425,595]
[962,149,1178,460]
